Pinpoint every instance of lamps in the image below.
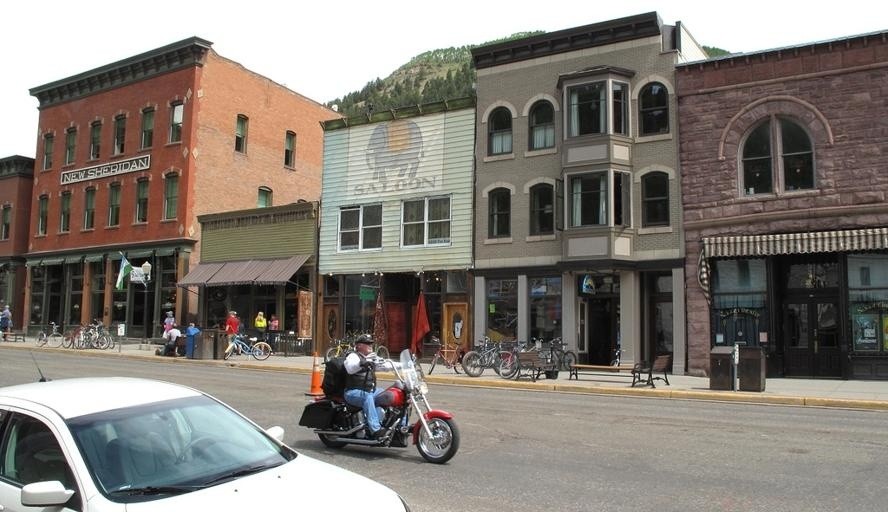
[416,265,424,276]
[374,270,384,277]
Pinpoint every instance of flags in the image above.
[372,291,388,347]
[411,293,430,353]
[115,256,133,290]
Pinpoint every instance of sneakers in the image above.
[370,428,386,439]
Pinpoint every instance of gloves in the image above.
[360,361,375,372]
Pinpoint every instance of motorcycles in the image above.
[299,346,460,464]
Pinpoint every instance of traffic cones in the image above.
[305,352,324,395]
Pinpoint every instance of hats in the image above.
[357,334,374,343]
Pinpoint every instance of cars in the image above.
[0,378,409,512]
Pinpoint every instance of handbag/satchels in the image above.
[322,356,347,396]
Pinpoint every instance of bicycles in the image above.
[63,320,115,349]
[610,349,621,371]
[36,321,64,348]
[224,331,273,360]
[428,336,576,378]
[324,330,367,364]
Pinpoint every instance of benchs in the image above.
[3,332,26,342]
[568,364,636,384]
[630,355,674,389]
[166,336,185,357]
[514,349,555,382]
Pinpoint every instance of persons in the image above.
[225,311,240,354]
[186,323,200,336]
[254,312,267,356]
[344,334,414,439]
[236,317,245,355]
[162,311,177,339]
[159,323,181,355]
[267,314,278,355]
[0,305,14,341]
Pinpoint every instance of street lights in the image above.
[141,260,152,344]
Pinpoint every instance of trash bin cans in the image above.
[215,330,230,359]
[710,346,735,390]
[193,329,215,359]
[186,327,202,358]
[738,345,767,391]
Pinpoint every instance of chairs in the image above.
[110,417,175,483]
